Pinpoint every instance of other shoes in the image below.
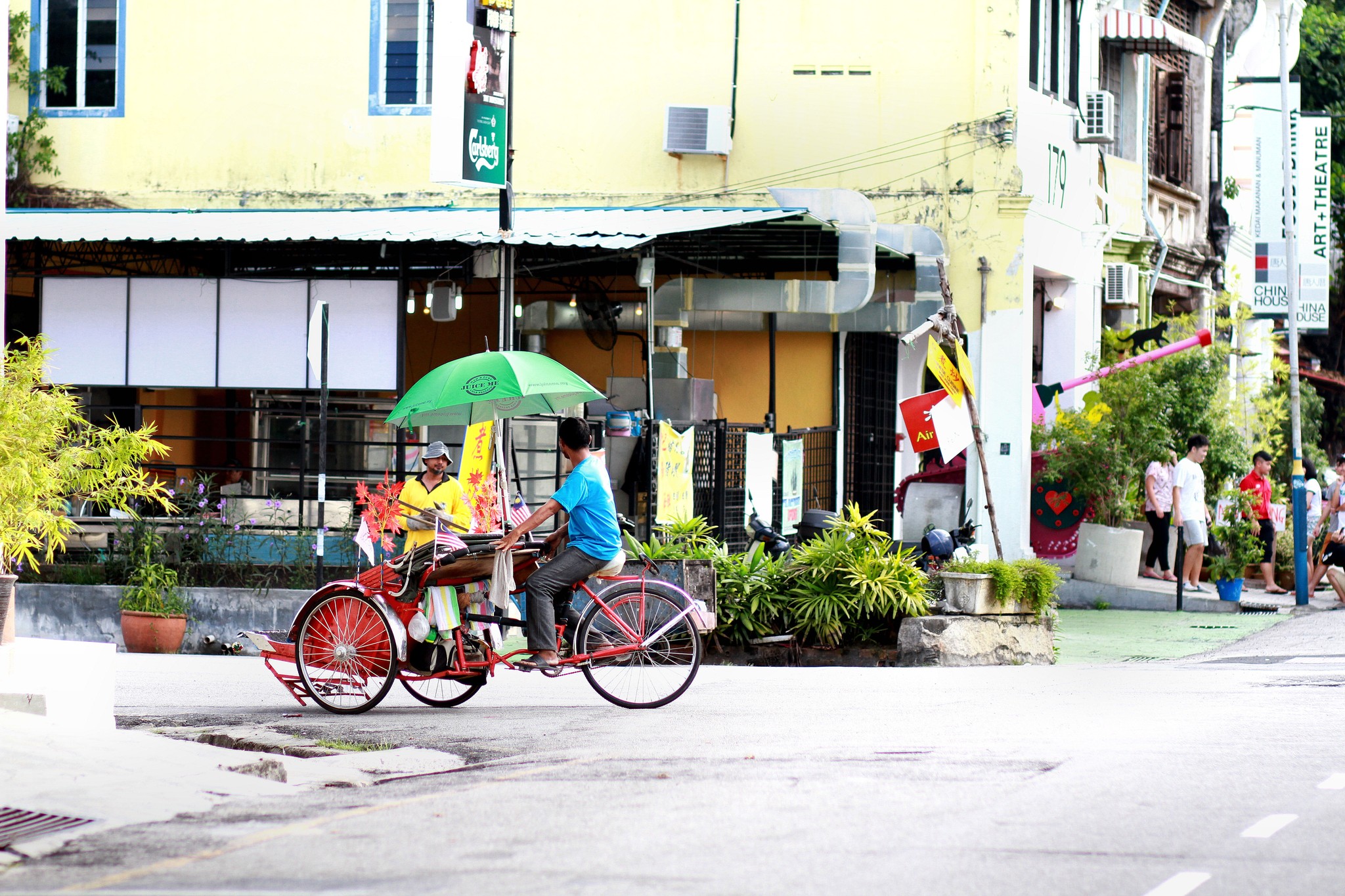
[1178,577,1199,592]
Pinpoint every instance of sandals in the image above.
[1143,570,1164,580]
[1326,601,1345,610]
[1162,575,1179,584]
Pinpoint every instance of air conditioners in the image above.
[1076,91,1115,142]
[662,103,733,156]
[1104,262,1139,307]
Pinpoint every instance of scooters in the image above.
[732,485,983,601]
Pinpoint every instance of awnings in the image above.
[5,206,810,250]
[1099,7,1206,58]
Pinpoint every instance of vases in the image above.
[1215,576,1245,601]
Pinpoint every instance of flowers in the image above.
[1210,490,1267,581]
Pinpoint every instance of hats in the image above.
[422,441,453,468]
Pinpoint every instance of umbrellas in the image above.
[383,336,608,531]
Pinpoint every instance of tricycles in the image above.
[238,528,706,716]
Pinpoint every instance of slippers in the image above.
[513,653,560,673]
[1290,589,1314,598]
[1263,586,1289,596]
[571,653,616,670]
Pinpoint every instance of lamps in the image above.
[406,282,523,319]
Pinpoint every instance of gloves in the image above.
[423,501,453,527]
[405,514,437,531]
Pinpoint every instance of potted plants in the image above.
[116,528,193,653]
[0,325,183,647]
[939,547,1066,626]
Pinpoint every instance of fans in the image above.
[573,277,647,364]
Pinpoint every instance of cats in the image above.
[1117,321,1171,356]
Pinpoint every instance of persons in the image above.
[1290,457,1345,597]
[1319,454,1345,563]
[1240,451,1288,594]
[1144,443,1179,582]
[490,416,622,671]
[1322,528,1345,609]
[1291,457,1322,585]
[1172,435,1211,594]
[395,441,472,555]
